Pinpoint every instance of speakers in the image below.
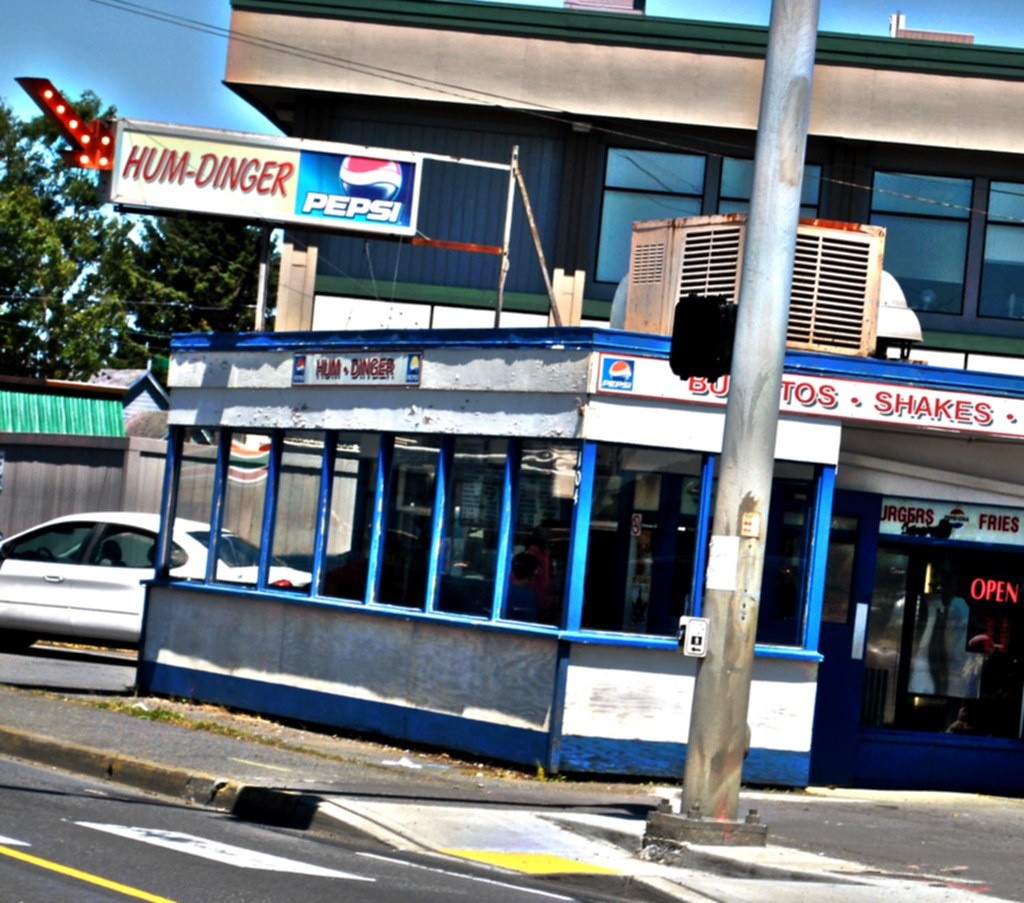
[670,292,738,382]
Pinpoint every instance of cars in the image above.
[0,510,314,655]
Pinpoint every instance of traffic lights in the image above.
[666,297,737,379]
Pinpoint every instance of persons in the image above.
[505,522,555,623]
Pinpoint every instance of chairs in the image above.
[98,539,130,567]
[144,542,156,569]
